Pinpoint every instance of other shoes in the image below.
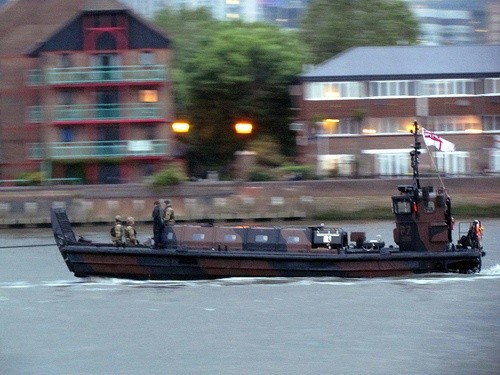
[150,245,158,248]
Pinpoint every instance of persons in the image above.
[152,200,176,246]
[110,215,140,248]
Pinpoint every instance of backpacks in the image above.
[110,226,115,237]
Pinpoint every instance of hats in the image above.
[127,217,134,224]
[115,215,122,221]
[164,200,170,205]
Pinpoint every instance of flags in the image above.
[421,126,455,152]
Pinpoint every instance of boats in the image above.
[48,119,488,284]
